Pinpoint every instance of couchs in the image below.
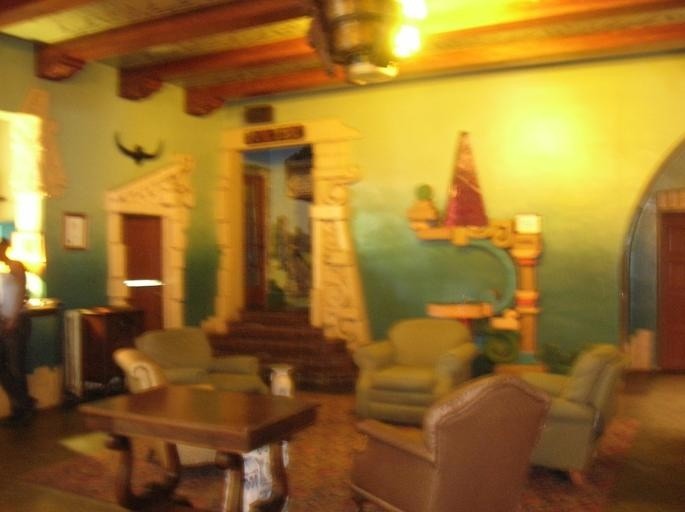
[353,371,552,512]
[511,344,628,483]
[352,318,478,424]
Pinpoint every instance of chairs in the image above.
[113,326,269,395]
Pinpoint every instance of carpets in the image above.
[22,389,640,511]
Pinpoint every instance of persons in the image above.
[0,234,40,430]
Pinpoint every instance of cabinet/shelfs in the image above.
[66,308,145,398]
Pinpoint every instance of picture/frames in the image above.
[63,211,88,249]
